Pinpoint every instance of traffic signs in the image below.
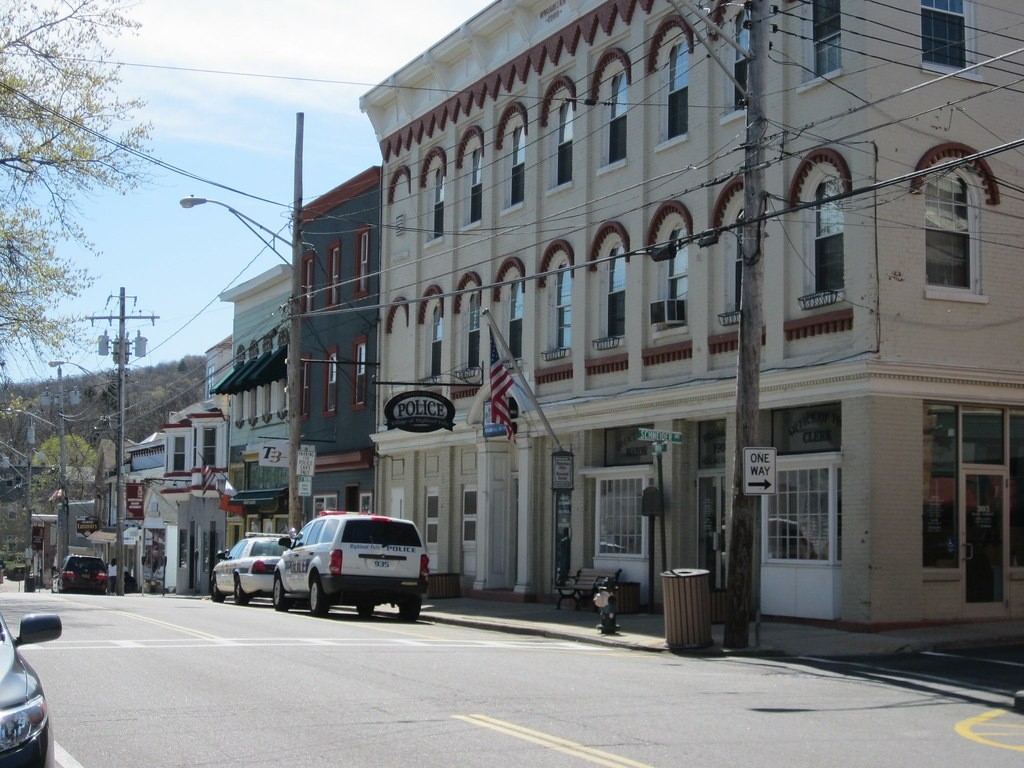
[742,447,777,495]
[638,428,683,445]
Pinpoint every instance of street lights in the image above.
[179,193,303,534]
[48,360,126,597]
[6,407,70,572]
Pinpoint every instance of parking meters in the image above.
[193,550,199,596]
[162,556,167,596]
[141,556,146,597]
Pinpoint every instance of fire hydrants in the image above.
[593,576,620,635]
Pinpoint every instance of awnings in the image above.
[85,532,116,543]
[228,487,288,505]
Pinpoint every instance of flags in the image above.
[488,326,516,447]
[218,480,243,517]
[201,459,216,495]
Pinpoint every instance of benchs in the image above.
[553,567,624,613]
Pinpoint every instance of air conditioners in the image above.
[649,299,686,326]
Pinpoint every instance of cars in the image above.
[209,531,296,605]
[0,606,62,768]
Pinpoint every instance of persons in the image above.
[106,558,117,595]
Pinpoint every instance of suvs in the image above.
[272,509,430,622]
[50,555,108,594]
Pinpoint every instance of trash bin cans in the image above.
[24,573,35,592]
[660,569,713,650]
